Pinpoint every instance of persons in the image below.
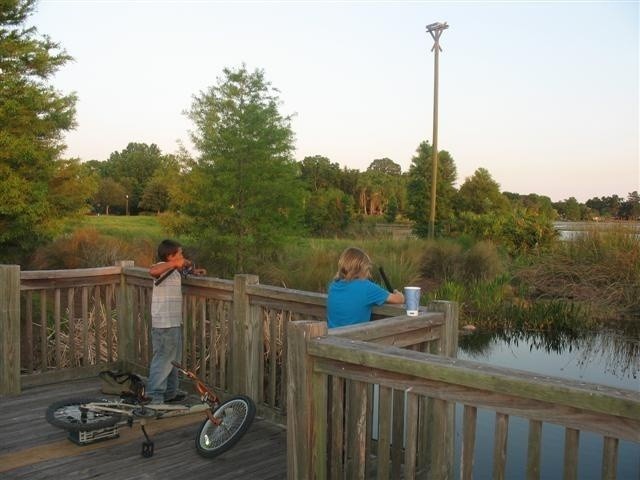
[324,246,405,467]
[147,237,207,404]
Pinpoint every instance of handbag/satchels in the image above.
[99,369,141,396]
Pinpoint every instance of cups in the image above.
[404,286,422,317]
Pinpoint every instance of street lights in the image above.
[427,22,449,240]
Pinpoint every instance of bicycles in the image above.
[42,360,256,458]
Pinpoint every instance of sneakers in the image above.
[123,394,153,405]
[168,390,189,402]
